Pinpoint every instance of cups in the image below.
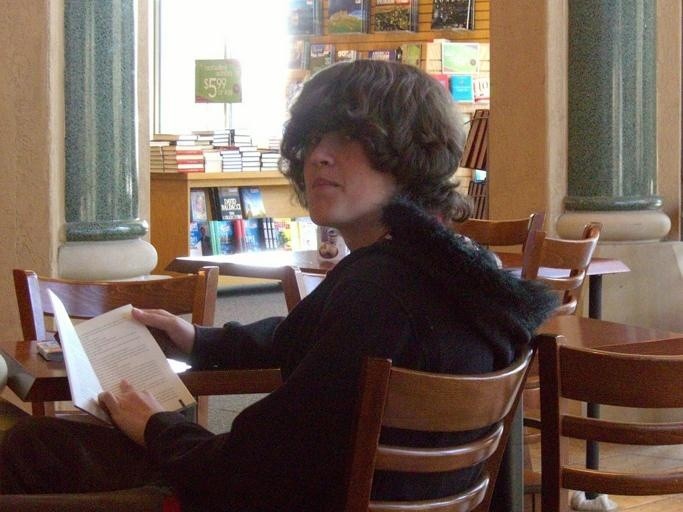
[317,221,347,263]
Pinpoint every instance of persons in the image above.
[0,60,560,512]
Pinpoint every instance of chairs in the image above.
[520,221,601,315]
[13,266,219,428]
[347,347,536,512]
[282,267,325,314]
[447,211,546,286]
[538,332,683,512]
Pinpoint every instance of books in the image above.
[44,287,197,431]
[430,73,490,220]
[36,340,65,363]
[188,186,319,256]
[150,128,287,173]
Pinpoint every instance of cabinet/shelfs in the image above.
[150,170,315,290]
